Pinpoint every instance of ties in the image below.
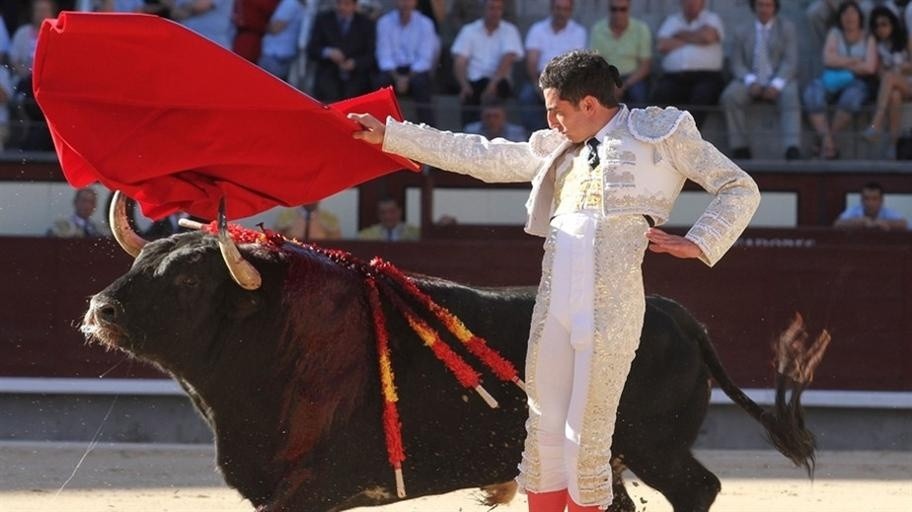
[586,137,599,167]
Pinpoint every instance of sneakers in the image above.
[824,147,840,160]
[735,146,750,158]
[885,145,898,162]
[786,145,799,159]
[862,126,880,142]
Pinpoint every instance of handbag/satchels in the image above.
[821,68,853,93]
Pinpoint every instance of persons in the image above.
[347,52,762,512]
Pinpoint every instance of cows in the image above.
[70,190,832,512]
